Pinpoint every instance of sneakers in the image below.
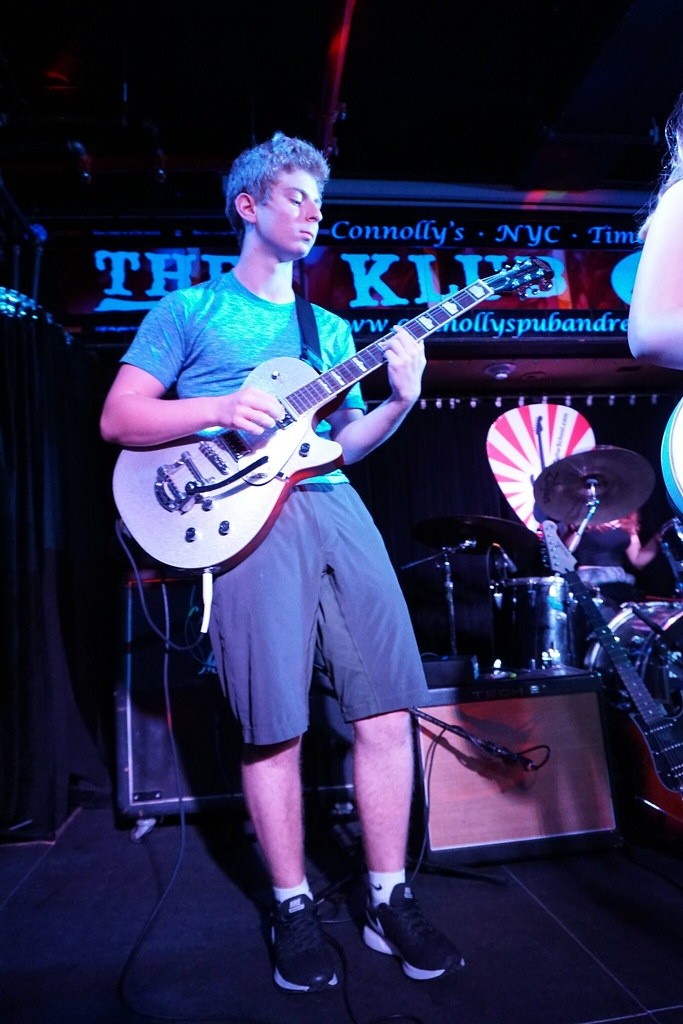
[360,883,465,984]
[268,894,340,996]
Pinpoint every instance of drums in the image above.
[581,601,682,727]
[488,574,635,668]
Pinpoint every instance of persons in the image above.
[629,161,683,371]
[98,133,466,993]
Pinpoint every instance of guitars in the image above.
[540,519,683,841]
[111,257,553,575]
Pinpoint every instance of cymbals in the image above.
[533,447,656,525]
[414,514,539,556]
